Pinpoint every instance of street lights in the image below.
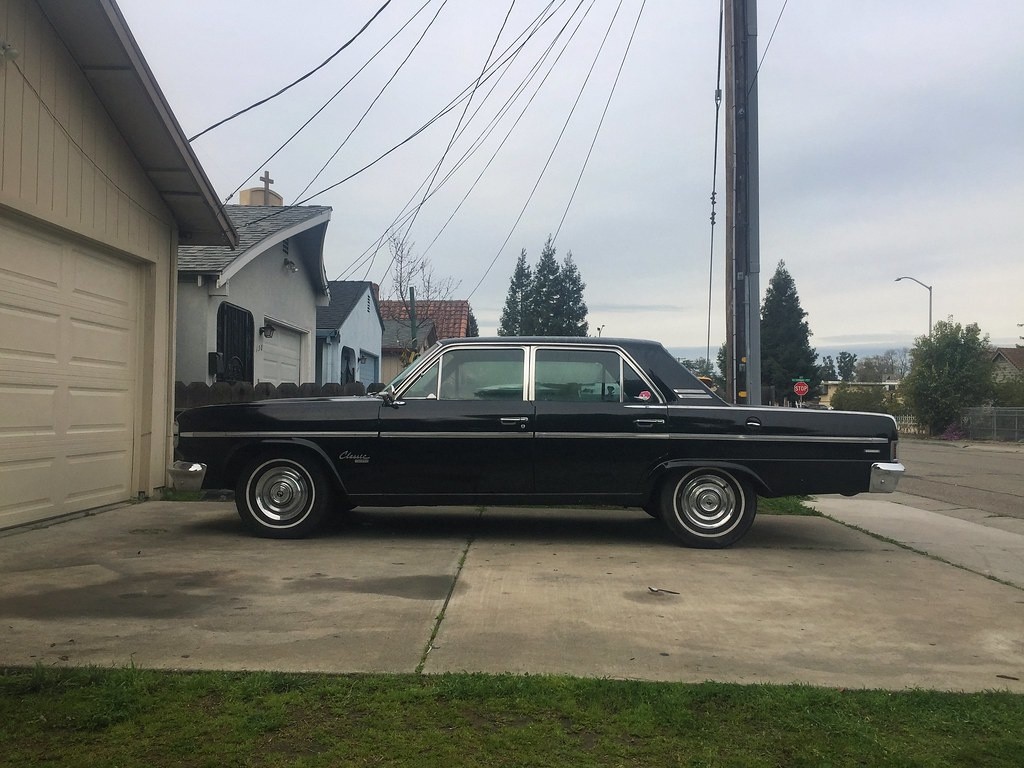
[596,325,605,337]
[894,277,933,340]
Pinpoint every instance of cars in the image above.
[173,333,905,549]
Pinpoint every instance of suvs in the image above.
[805,404,828,410]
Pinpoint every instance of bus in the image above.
[696,376,717,394]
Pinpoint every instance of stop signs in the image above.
[794,382,808,396]
